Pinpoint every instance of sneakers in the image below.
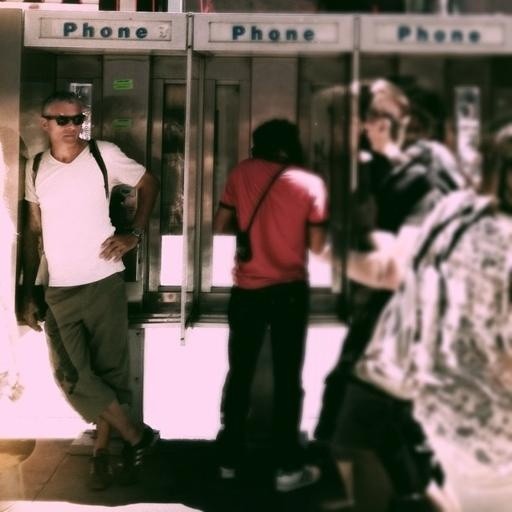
[85,447,112,491]
[118,424,159,484]
[267,464,326,493]
[211,458,237,480]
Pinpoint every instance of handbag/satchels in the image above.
[233,230,254,261]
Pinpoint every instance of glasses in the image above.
[40,110,85,129]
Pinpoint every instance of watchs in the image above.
[128,229,143,245]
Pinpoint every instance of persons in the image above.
[210,120,327,496]
[409,126,512,512]
[322,79,466,511]
[25,91,162,493]
